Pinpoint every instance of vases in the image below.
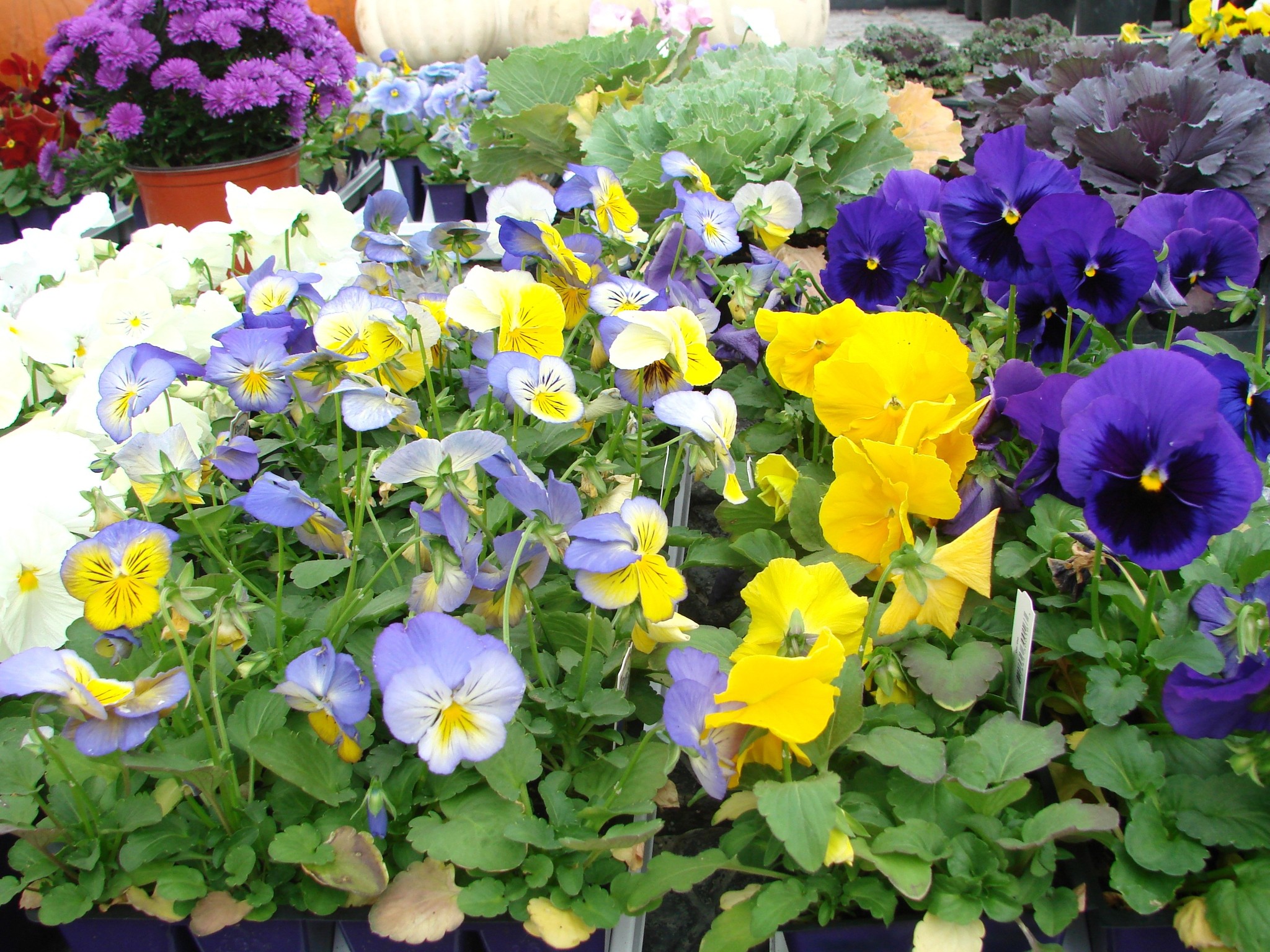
[124,133,304,235]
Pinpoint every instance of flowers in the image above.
[37,0,356,197]
